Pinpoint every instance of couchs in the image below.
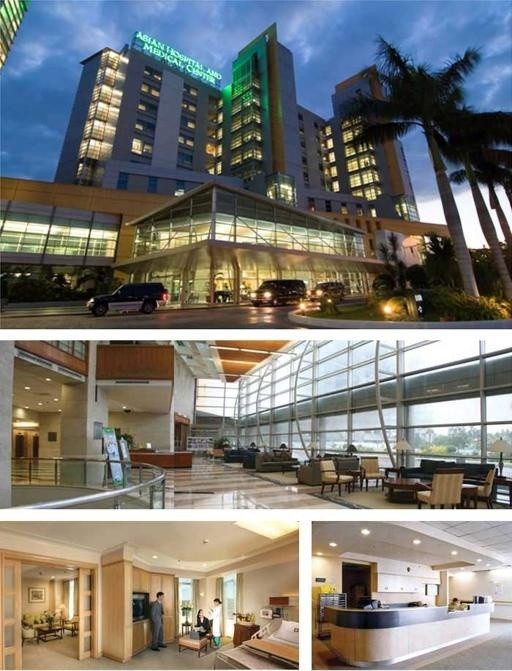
[224,448,255,469]
[23,612,74,634]
[398,458,498,503]
[296,456,360,486]
[255,451,299,472]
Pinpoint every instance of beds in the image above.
[281,464,300,477]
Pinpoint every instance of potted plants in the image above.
[181,607,191,625]
[39,611,57,628]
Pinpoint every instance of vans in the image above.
[250,280,306,307]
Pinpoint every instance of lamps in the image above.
[392,436,413,466]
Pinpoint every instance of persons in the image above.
[150,592,167,651]
[194,608,209,637]
[448,597,466,613]
[209,599,223,648]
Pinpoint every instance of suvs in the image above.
[309,282,344,303]
[86,282,168,317]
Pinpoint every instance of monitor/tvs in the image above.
[133,592,149,625]
[359,596,371,608]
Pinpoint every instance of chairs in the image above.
[319,457,386,497]
[205,620,213,648]
[413,465,497,509]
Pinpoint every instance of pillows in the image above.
[24,613,47,625]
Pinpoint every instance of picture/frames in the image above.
[27,587,46,603]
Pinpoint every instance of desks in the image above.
[233,623,260,647]
